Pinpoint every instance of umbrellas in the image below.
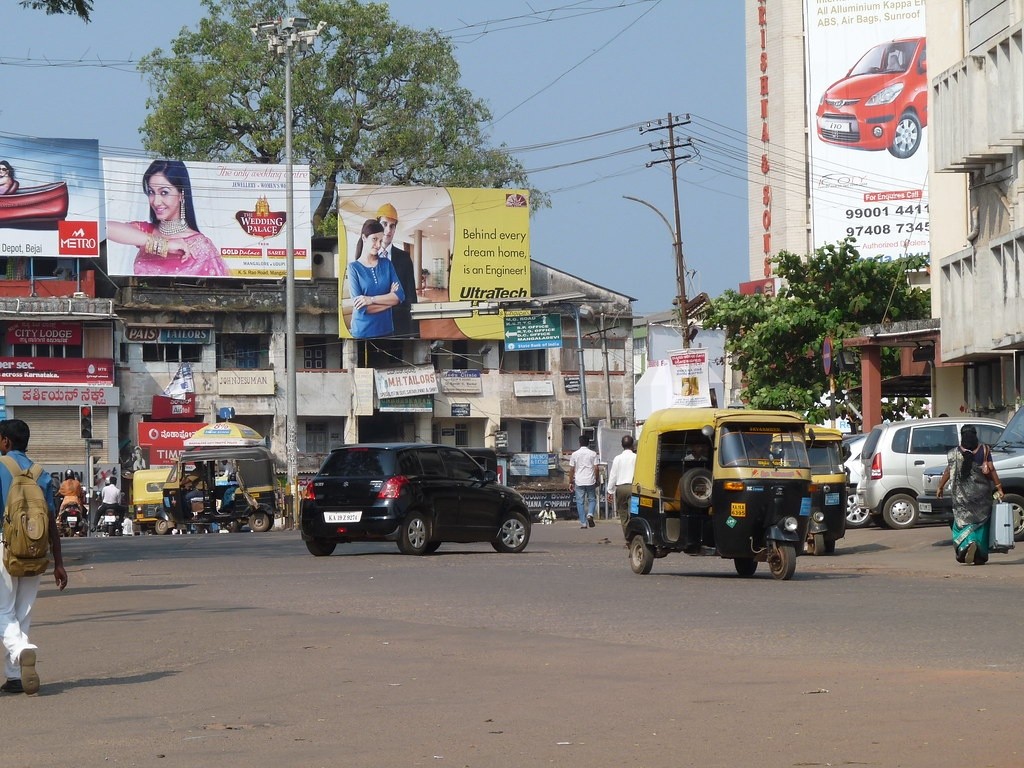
[183,421,266,447]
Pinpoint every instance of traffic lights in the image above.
[79,405,93,438]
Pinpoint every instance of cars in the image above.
[917,405,1024,542]
[839,434,882,529]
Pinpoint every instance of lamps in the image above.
[430,339,445,351]
[478,345,493,355]
[53,267,64,276]
[912,345,936,362]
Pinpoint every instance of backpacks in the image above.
[0,456,50,578]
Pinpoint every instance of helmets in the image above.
[105,477,110,486]
[544,503,551,509]
[375,203,398,224]
[65,469,74,479]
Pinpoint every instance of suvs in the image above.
[816,37,928,159]
[298,442,533,555]
[861,417,1024,528]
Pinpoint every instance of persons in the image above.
[606,435,637,536]
[179,461,208,519]
[348,219,405,339]
[568,434,600,529]
[0,419,68,695]
[681,443,708,461]
[375,202,420,339]
[54,469,88,528]
[133,446,146,470]
[936,424,1004,565]
[106,159,234,277]
[537,502,557,524]
[90,476,133,537]
[0,160,15,195]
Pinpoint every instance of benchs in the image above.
[190,496,208,512]
[220,487,237,512]
[660,464,681,512]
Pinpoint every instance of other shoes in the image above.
[19,648,41,695]
[974,558,985,564]
[587,515,595,527]
[581,527,587,529]
[965,543,977,563]
[0,679,22,692]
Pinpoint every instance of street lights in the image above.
[530,300,589,427]
[579,304,613,429]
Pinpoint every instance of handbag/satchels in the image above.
[981,445,994,480]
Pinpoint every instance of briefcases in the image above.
[988,497,1015,554]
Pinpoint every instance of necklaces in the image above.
[158,217,190,235]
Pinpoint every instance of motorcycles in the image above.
[58,493,86,537]
[537,516,554,525]
[96,491,126,537]
[625,407,815,580]
[132,468,174,534]
[154,446,281,535]
[768,423,852,557]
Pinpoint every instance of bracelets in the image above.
[995,483,1002,487]
[144,232,169,258]
